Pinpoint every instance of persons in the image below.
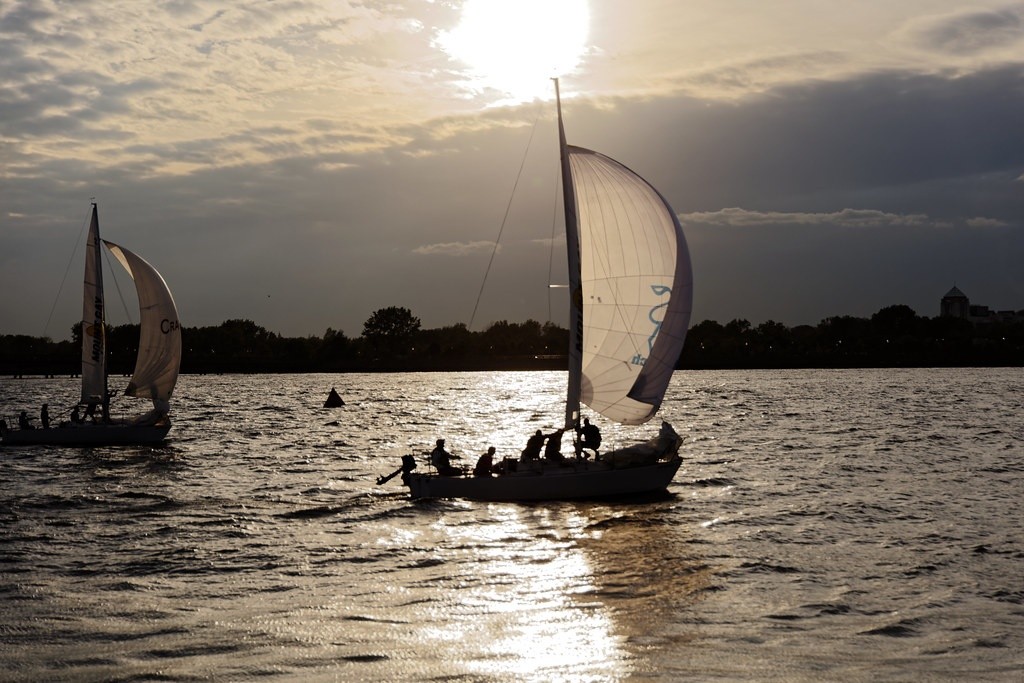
[82,403,97,421]
[521,429,565,461]
[430,439,462,477]
[18,412,39,429]
[473,447,496,476]
[574,418,601,460]
[71,405,79,422]
[41,404,49,429]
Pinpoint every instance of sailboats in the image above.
[374,77,686,508]
[0,194,185,448]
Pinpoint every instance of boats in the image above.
[534,347,569,370]
